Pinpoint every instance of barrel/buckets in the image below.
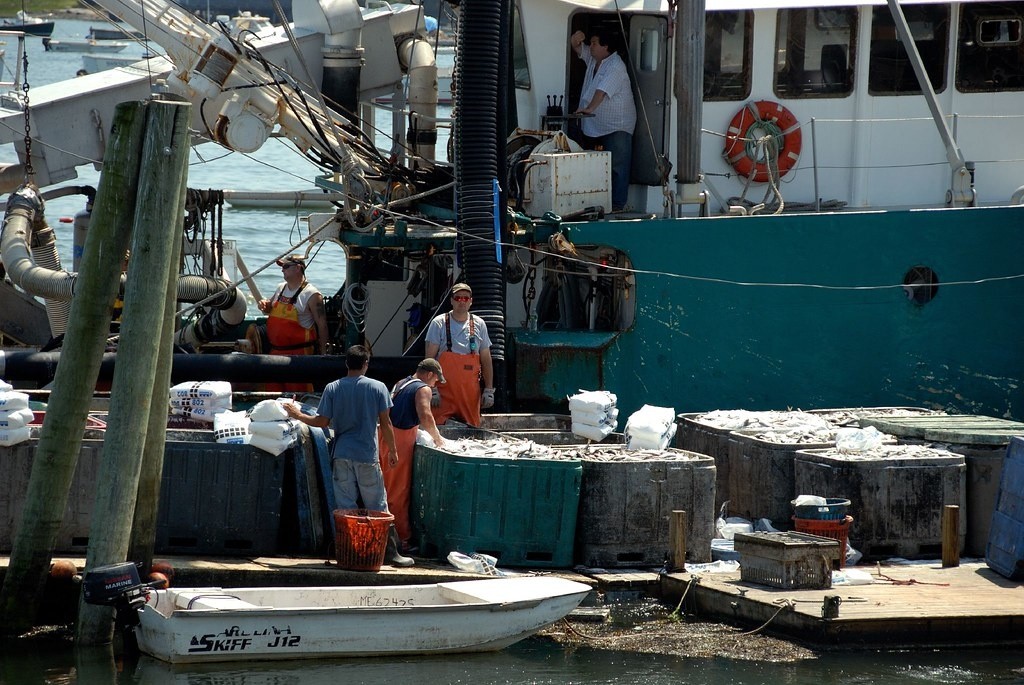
[334,509,394,571]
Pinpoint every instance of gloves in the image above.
[481,388,496,408]
[430,386,441,408]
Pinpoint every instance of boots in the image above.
[385,525,416,567]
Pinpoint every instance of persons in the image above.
[257,256,330,393]
[425,283,495,426]
[378,358,446,555]
[281,345,415,567]
[571,31,637,213]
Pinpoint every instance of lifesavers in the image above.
[727,101,802,182]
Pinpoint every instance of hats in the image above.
[418,358,446,382]
[452,283,471,294]
[277,255,305,274]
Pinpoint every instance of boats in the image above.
[83,561,594,666]
[2,1,1024,434]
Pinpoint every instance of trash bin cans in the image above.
[790,495,850,572]
[334,507,394,571]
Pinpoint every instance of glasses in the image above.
[453,296,472,302]
[282,265,296,269]
